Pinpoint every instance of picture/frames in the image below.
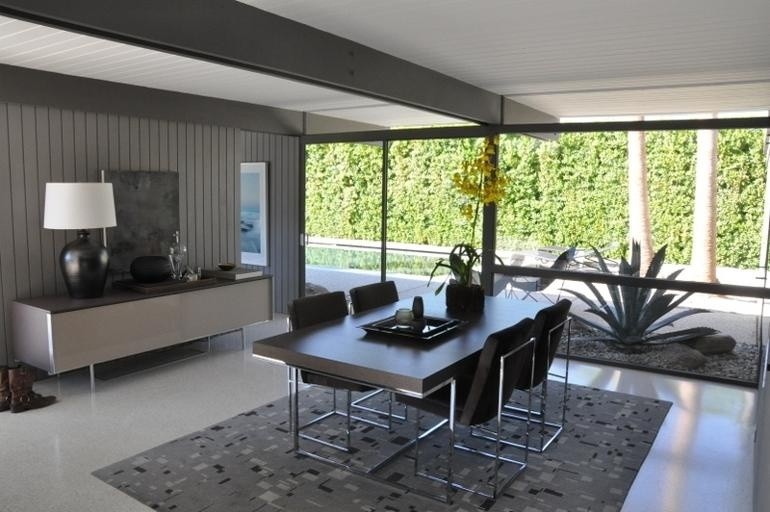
[239,162,268,268]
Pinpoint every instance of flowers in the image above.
[426,132,513,297]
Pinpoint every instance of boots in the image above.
[10,364,57,413]
[0,364,13,413]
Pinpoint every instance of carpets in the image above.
[90,364,674,512]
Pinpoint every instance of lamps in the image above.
[43,180,119,300]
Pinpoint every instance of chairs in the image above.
[467,302,572,452]
[408,319,529,498]
[288,292,393,453]
[450,244,619,305]
[347,280,429,421]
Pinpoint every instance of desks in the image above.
[10,255,274,387]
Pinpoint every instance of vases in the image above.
[440,285,497,322]
